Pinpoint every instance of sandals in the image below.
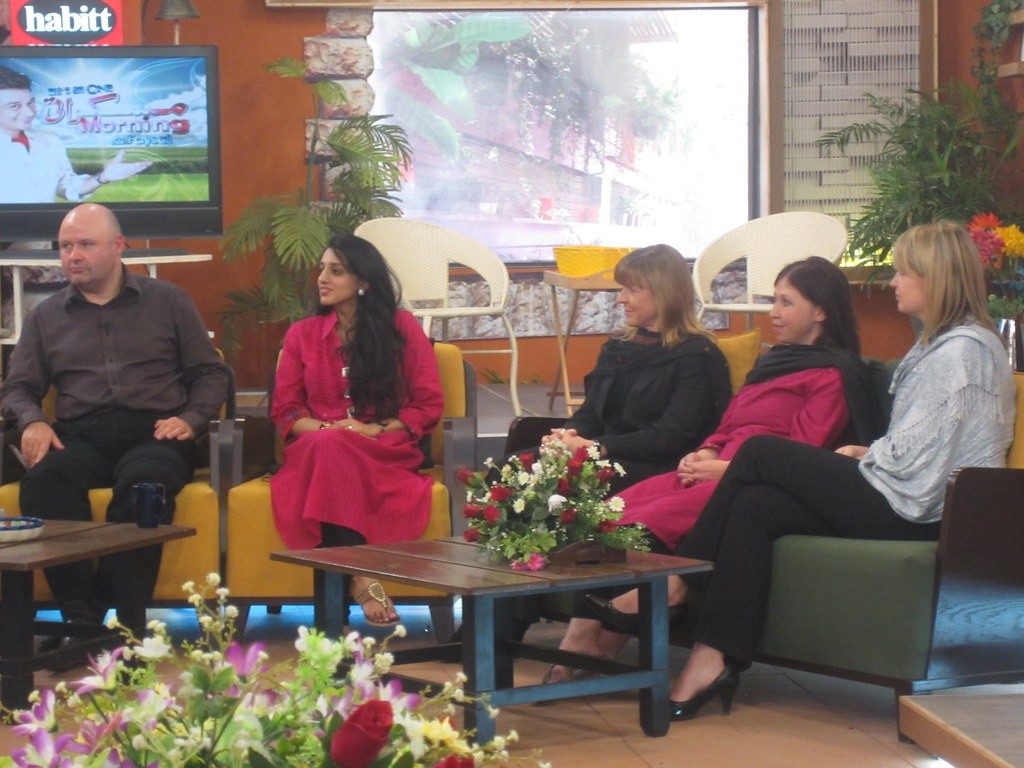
[350,580,400,627]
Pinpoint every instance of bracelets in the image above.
[319,422,330,429]
[96,175,104,184]
[594,439,601,452]
[378,424,384,432]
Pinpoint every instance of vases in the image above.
[997,318,1024,374]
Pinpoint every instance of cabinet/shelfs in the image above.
[544,271,625,414]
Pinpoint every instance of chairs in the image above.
[0,348,230,651]
[354,216,520,418]
[690,211,847,334]
[223,340,478,646]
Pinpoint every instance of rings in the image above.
[349,426,352,430]
[561,429,565,434]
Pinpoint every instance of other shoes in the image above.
[536,652,622,682]
[36,619,91,648]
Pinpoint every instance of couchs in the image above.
[506,376,1023,743]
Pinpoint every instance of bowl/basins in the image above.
[0,517,44,542]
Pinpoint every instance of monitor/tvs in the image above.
[0,46,223,260]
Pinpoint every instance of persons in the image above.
[455,243,732,640]
[268,233,445,646]
[542,256,871,685]
[0,203,234,665]
[0,65,153,204]
[585,220,1015,719]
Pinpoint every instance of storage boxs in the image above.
[553,247,636,279]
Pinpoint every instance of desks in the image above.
[0,520,195,719]
[269,535,715,748]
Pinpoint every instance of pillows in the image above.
[711,327,761,395]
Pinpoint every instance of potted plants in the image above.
[214,56,413,421]
[815,76,1019,346]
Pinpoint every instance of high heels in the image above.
[669,661,740,720]
[585,591,688,632]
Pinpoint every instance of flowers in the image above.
[459,435,653,573]
[967,213,1024,317]
[0,574,553,768]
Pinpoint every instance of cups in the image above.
[133,483,167,531]
